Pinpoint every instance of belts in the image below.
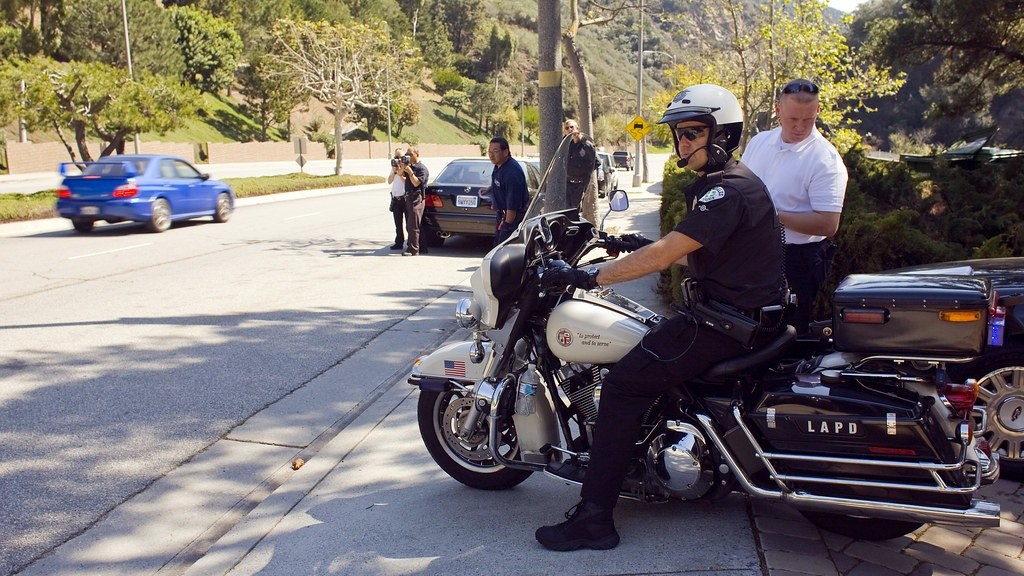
[392,196,405,201]
[406,190,418,196]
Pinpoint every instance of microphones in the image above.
[677,144,709,169]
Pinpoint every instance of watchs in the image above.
[587,267,599,286]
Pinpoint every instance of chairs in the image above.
[463,172,480,182]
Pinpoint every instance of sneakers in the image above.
[535,501,620,552]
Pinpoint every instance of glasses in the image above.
[405,153,417,157]
[674,124,712,141]
[394,157,402,160]
[778,83,818,101]
[488,149,504,156]
[564,125,575,130]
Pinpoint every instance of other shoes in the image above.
[402,251,419,255]
[390,243,404,250]
[420,248,429,254]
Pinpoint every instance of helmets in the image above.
[656,84,743,154]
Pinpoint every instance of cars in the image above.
[57,154,235,234]
[611,151,636,172]
[415,157,540,247]
[596,152,621,200]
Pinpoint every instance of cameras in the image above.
[401,155,410,165]
[391,156,402,168]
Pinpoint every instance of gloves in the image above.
[620,233,654,253]
[533,259,598,294]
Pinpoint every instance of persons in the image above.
[535,84,790,551]
[388,138,529,256]
[561,120,601,210]
[740,78,848,335]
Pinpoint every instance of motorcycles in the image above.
[405,130,1005,542]
[800,263,1024,477]
